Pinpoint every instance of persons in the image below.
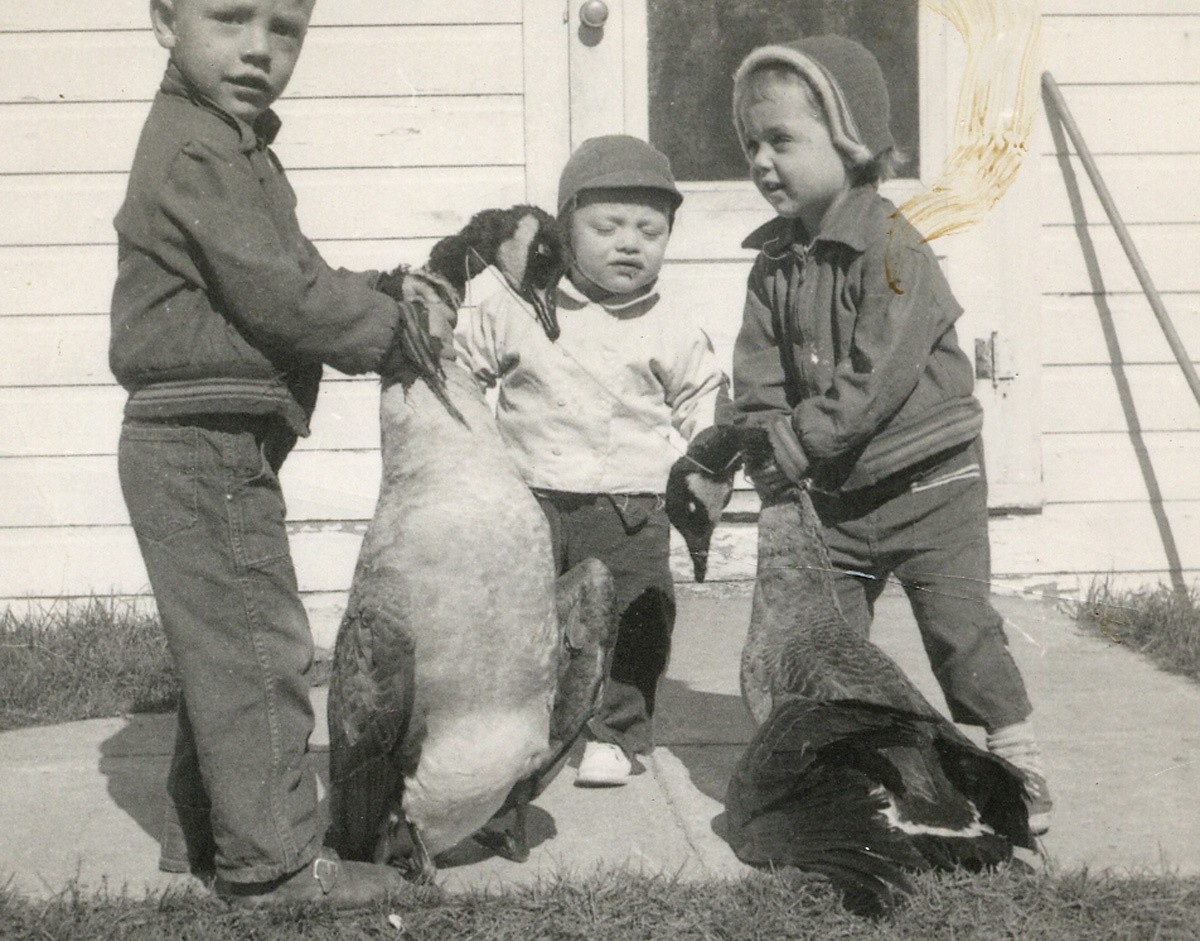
[731,34,1053,833]
[446,136,738,785]
[110,0,457,908]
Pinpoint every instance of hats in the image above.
[555,134,683,219]
[732,36,895,165]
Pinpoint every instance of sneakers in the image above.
[575,740,631,786]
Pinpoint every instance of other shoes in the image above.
[1016,767,1052,836]
[160,804,217,874]
[229,856,402,917]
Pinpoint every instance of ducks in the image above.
[321,202,570,889]
[664,425,1036,912]
